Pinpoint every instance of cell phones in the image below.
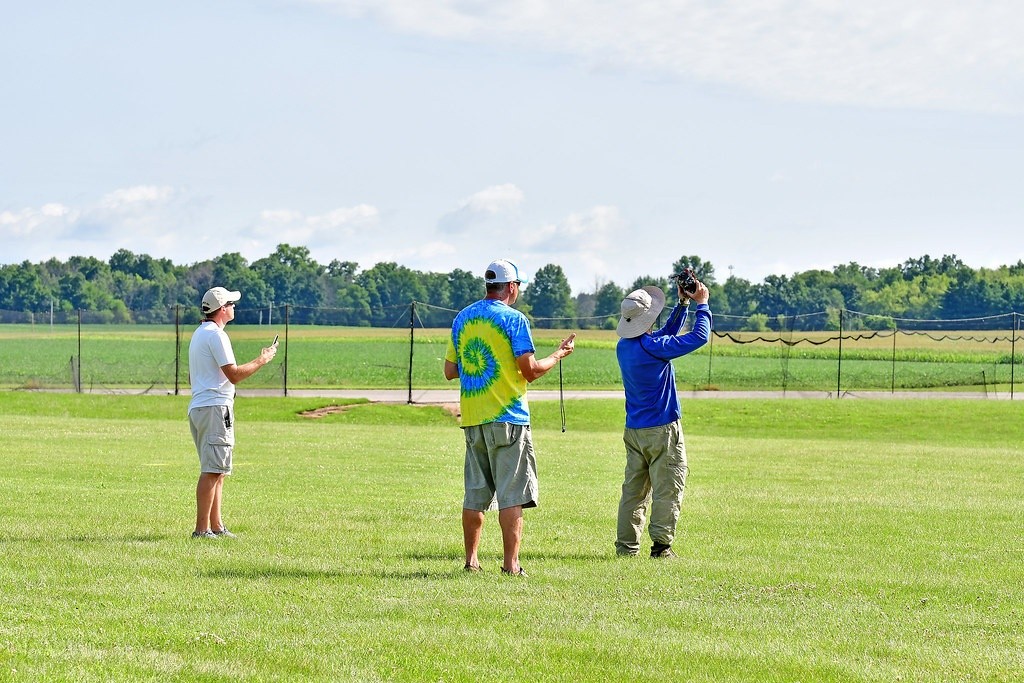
[273,334,279,345]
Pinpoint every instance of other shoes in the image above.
[463,564,484,572]
[211,526,238,538]
[500,566,529,578]
[614,540,641,557]
[650,544,684,559]
[191,528,219,538]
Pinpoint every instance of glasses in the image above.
[225,301,235,307]
[513,281,520,286]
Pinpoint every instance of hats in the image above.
[615,286,665,338]
[201,286,242,313]
[483,258,529,284]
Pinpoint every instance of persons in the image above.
[444,259,576,578]
[186,287,279,538]
[614,278,713,558]
